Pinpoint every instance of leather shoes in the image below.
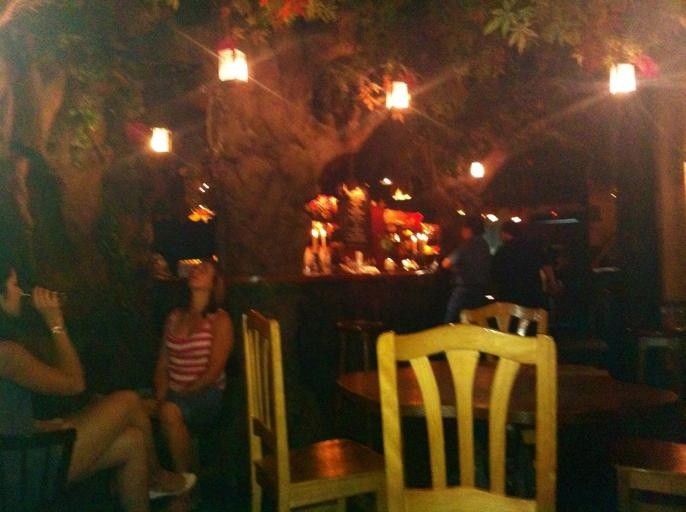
[149,471,197,500]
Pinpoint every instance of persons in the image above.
[149,259,238,511]
[0,251,199,512]
[489,220,528,303]
[441,213,491,327]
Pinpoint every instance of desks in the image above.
[338,359,677,427]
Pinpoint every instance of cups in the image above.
[21,289,68,312]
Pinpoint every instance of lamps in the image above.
[609,63,636,92]
[385,81,409,109]
[216,7,250,82]
[149,120,171,153]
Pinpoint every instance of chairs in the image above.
[336,319,382,448]
[376,324,556,512]
[459,302,548,361]
[0,427,77,512]
[612,334,686,512]
[242,309,386,512]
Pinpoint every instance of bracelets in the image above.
[48,325,66,336]
